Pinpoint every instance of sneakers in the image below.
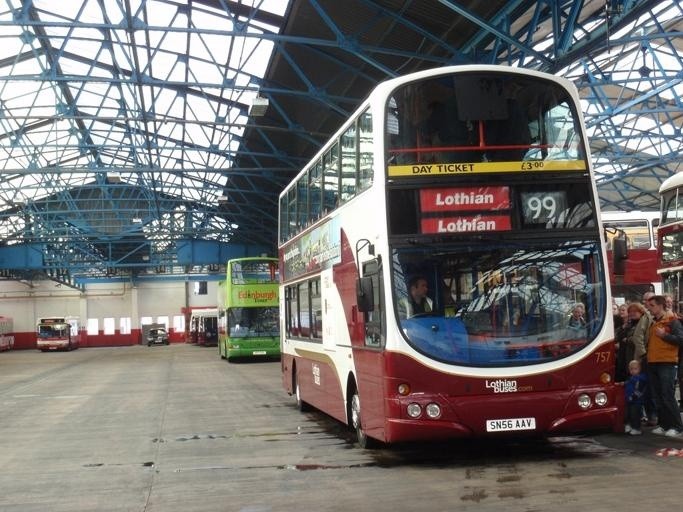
[625,417,682,438]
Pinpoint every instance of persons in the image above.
[398,277,433,321]
[611,292,683,437]
[566,302,586,330]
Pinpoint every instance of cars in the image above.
[145,327,170,348]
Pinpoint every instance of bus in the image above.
[272,61,628,449]
[183,308,218,348]
[216,257,280,362]
[598,171,682,321]
[35,316,80,352]
[0,315,14,353]
